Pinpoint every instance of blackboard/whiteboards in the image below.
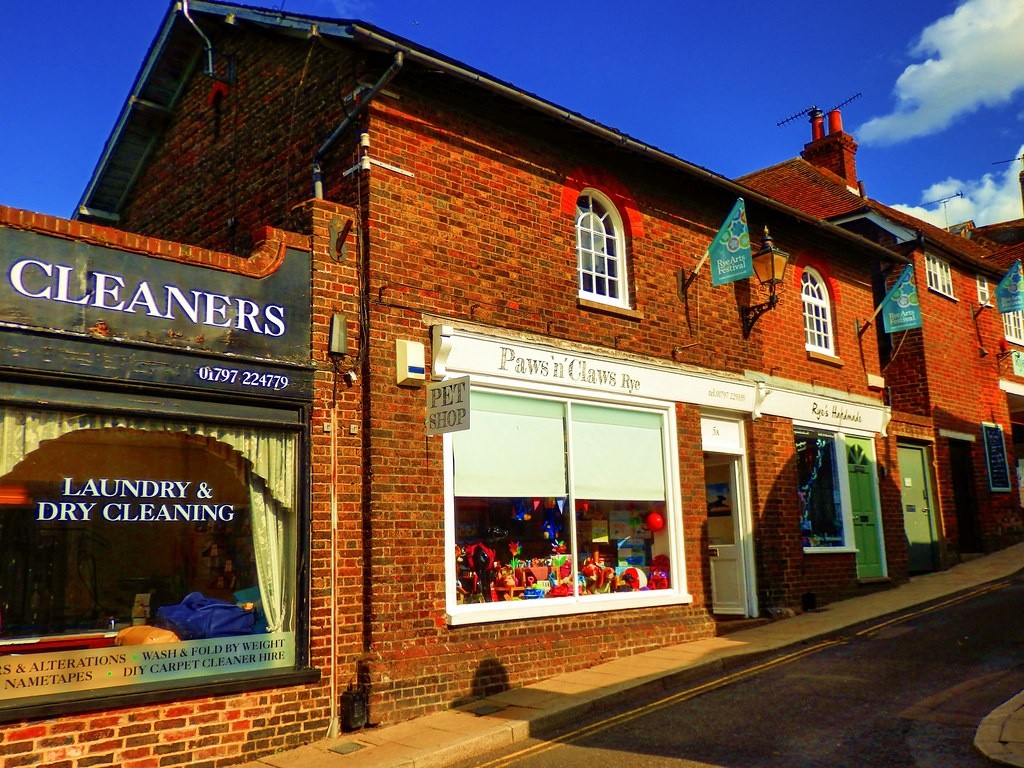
[982,422,1012,492]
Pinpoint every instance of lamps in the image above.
[740,225,790,339]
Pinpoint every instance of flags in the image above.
[881,264,922,333]
[995,258,1024,314]
[709,198,754,287]
